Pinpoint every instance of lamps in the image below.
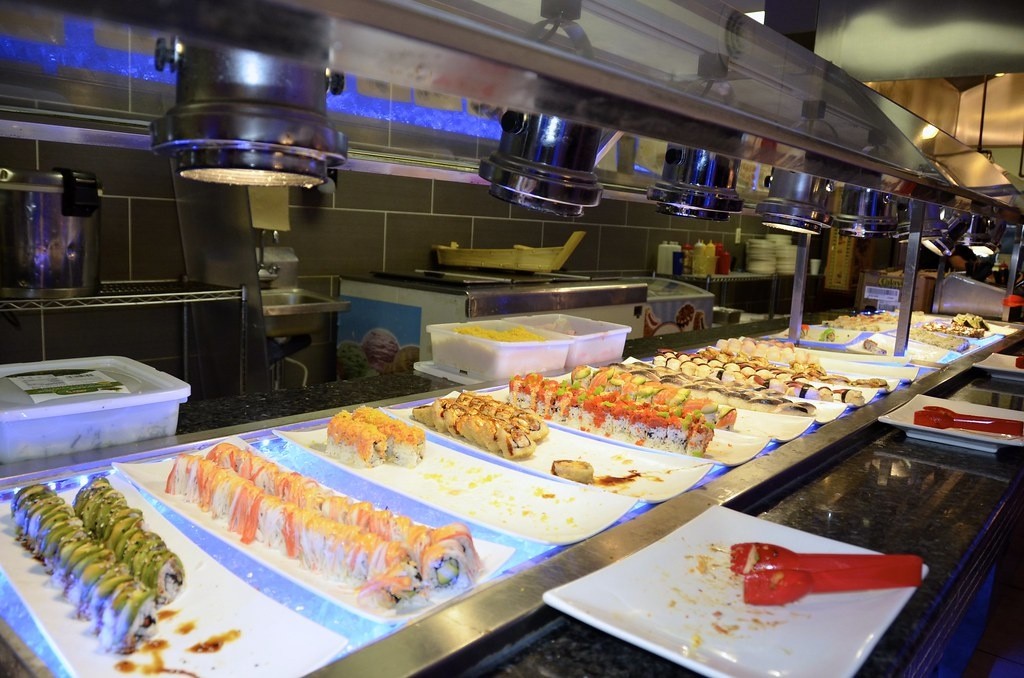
[891,196,950,246]
[955,207,991,246]
[477,108,604,217]
[752,165,836,234]
[922,205,973,256]
[838,183,897,240]
[145,104,351,190]
[968,216,1008,256]
[647,141,746,222]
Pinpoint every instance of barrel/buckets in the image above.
[260,288,352,391]
[1,163,102,302]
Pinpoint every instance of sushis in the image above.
[11,310,990,645]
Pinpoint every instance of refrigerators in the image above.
[339,270,646,379]
[590,276,716,339]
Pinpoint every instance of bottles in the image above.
[656,238,737,276]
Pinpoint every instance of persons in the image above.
[971,215,996,247]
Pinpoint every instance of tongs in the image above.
[730,542,923,606]
[914,405,1023,437]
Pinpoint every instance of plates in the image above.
[919,321,996,341]
[541,365,817,445]
[706,338,921,384]
[888,329,980,355]
[972,352,1024,381]
[376,390,715,503]
[945,318,1019,336]
[584,356,847,423]
[729,336,912,366]
[647,350,876,406]
[538,505,932,678]
[685,346,899,393]
[475,379,771,467]
[272,409,638,548]
[911,309,938,325]
[769,325,862,344]
[1,476,348,676]
[820,316,897,332]
[877,393,1024,453]
[746,233,798,275]
[110,435,517,625]
[845,332,949,363]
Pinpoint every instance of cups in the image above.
[810,259,821,275]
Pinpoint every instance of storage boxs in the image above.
[504,309,633,369]
[426,318,572,381]
[2,346,192,466]
